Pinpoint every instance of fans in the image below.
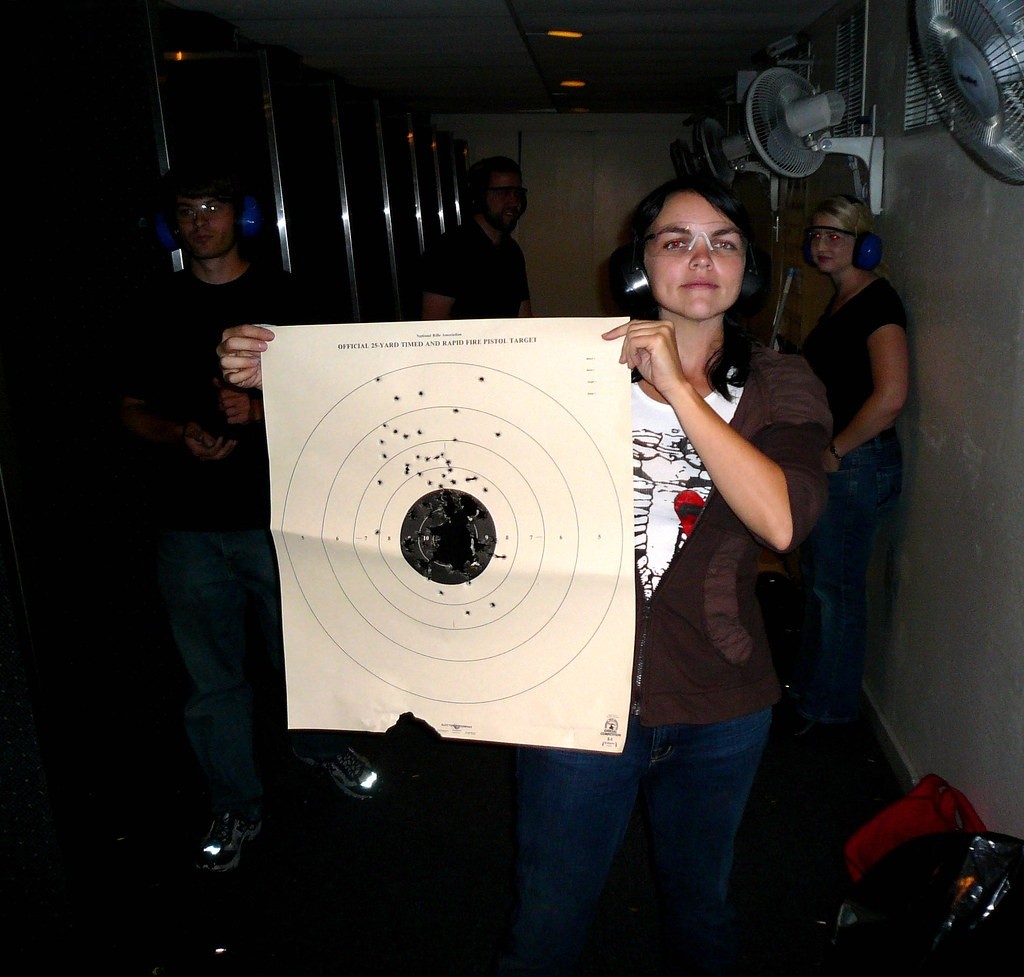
[744,67,885,216]
[912,0,1024,185]
[700,118,779,212]
[670,138,732,194]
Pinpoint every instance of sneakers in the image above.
[196,810,262,872]
[291,743,383,798]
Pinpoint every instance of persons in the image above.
[120,167,388,876]
[410,155,533,320]
[769,194,910,752]
[214,178,835,977]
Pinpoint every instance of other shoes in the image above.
[791,713,816,737]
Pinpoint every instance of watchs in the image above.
[826,441,843,462]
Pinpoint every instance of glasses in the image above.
[645,221,749,257]
[807,232,849,249]
[482,186,528,198]
[174,199,226,223]
